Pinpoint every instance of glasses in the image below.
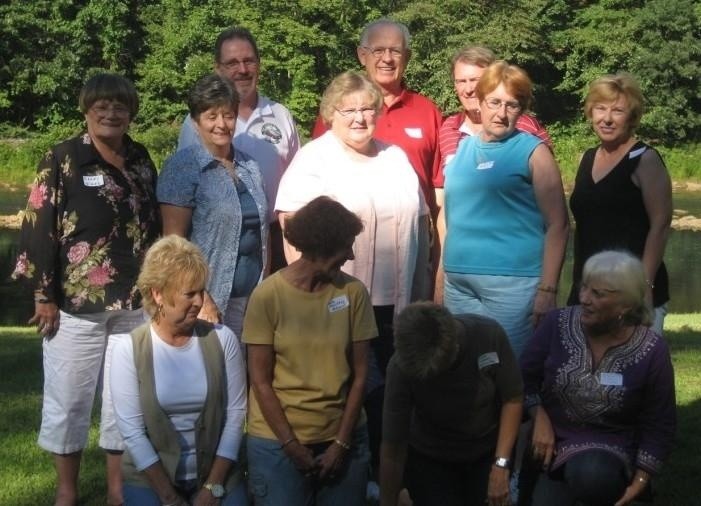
[483,97,521,113]
[335,107,377,116]
[362,45,406,58]
[219,59,261,69]
[90,104,133,119]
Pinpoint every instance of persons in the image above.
[107,236,248,505]
[377,301,527,505]
[313,19,448,311]
[270,68,432,502]
[434,46,562,190]
[441,62,567,506]
[8,72,163,506]
[571,73,673,339]
[156,75,279,360]
[515,249,677,506]
[177,26,302,281]
[241,196,382,504]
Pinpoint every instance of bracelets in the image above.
[645,277,658,289]
[334,439,354,453]
[280,437,299,448]
[537,285,560,296]
[30,289,55,302]
[634,475,650,483]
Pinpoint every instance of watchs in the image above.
[491,456,511,468]
[201,482,227,499]
